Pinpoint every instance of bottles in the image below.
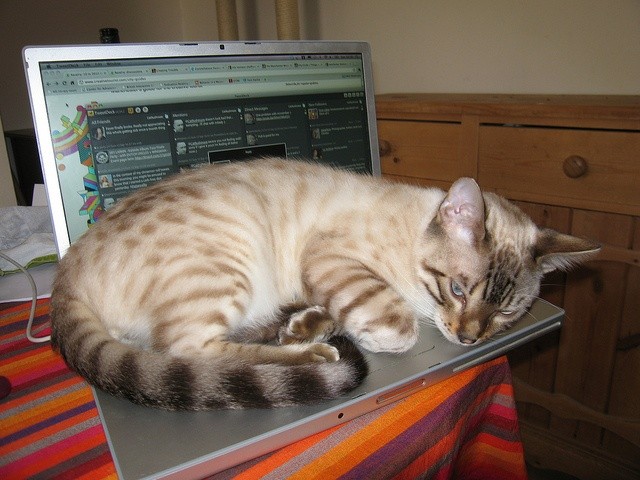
[99,27,120,43]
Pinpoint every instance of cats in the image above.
[49,156,602,411]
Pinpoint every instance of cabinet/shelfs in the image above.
[376,94,640,480]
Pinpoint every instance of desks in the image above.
[0,295,527,480]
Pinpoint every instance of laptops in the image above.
[21,40,566,480]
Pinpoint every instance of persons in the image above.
[177,142,186,155]
[100,175,110,188]
[104,198,114,211]
[311,112,316,119]
[174,120,183,132]
[247,135,255,145]
[92,126,105,140]
[245,114,253,124]
